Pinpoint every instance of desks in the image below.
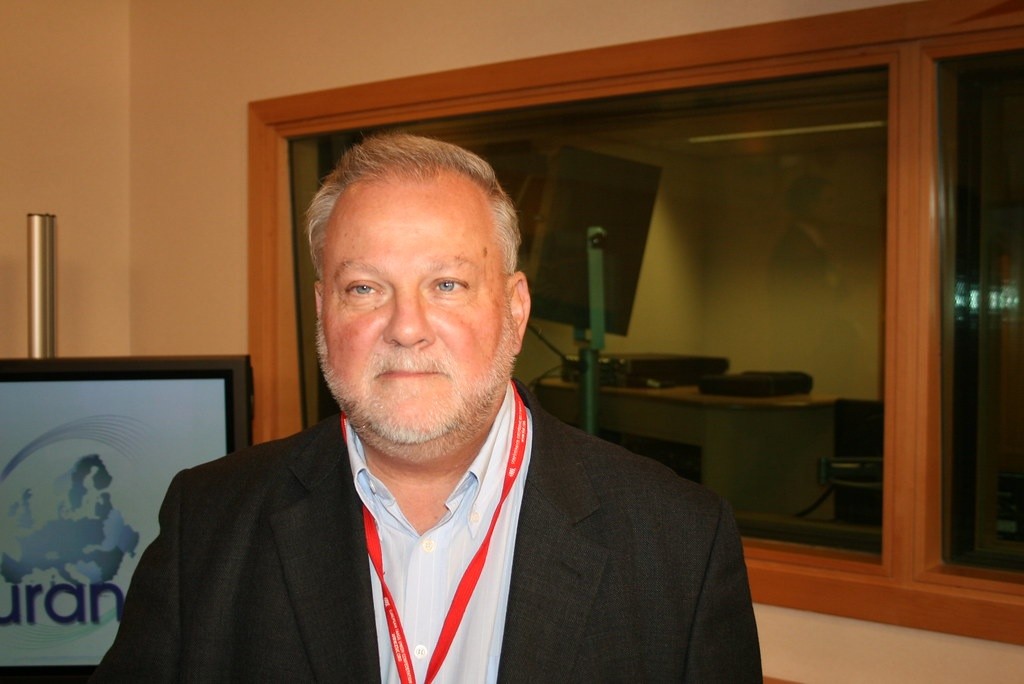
[538,377,843,524]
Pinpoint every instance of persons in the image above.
[94,134,764,684]
